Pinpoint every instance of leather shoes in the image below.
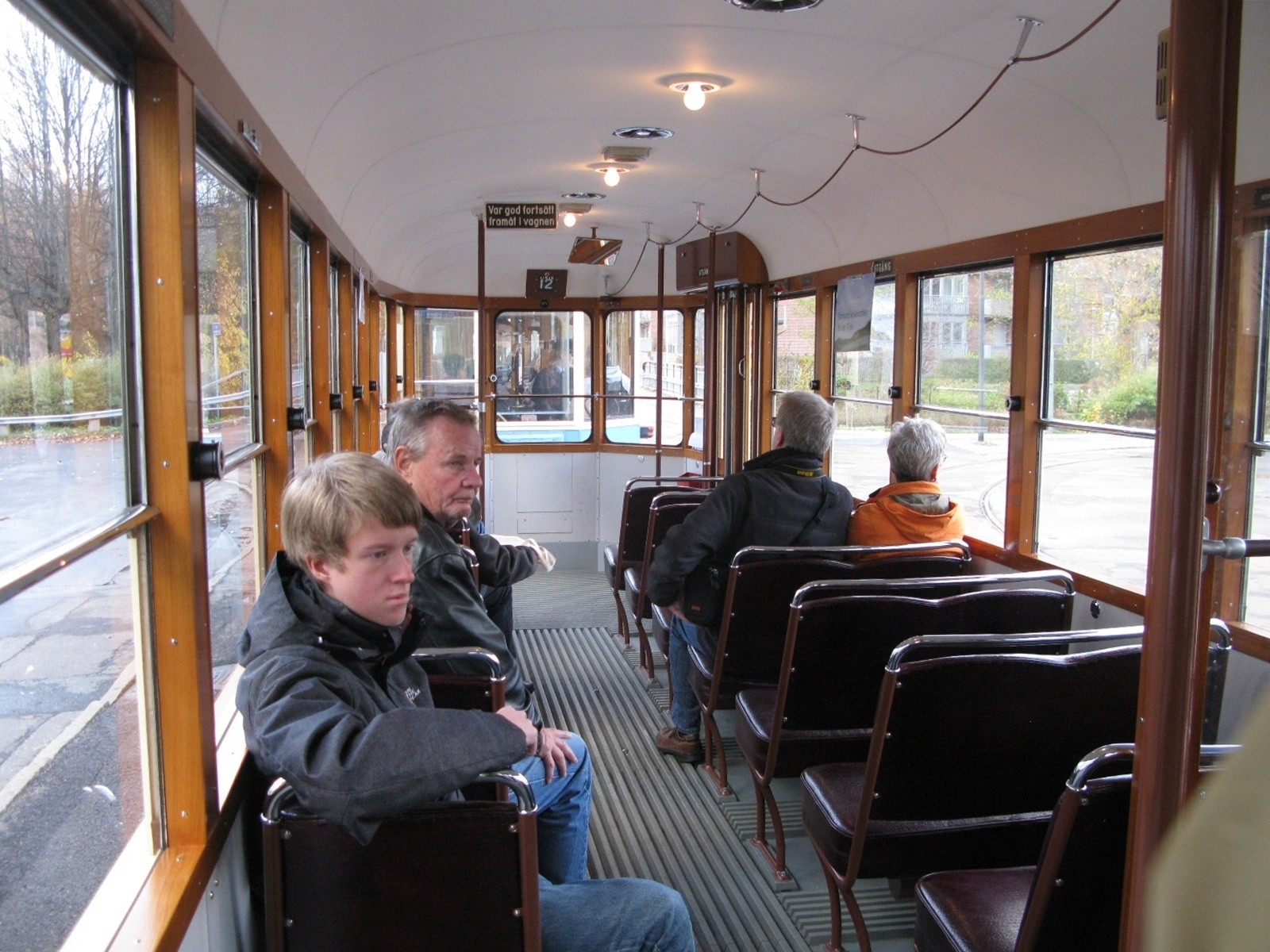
[655,728,704,762]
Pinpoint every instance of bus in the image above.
[380,307,655,445]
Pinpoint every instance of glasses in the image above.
[771,417,777,426]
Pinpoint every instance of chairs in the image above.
[259,515,540,952]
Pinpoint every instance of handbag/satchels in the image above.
[683,565,726,627]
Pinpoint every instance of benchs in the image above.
[602,475,1250,952]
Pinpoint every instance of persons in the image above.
[235,450,696,952]
[656,391,856,764]
[848,416,967,564]
[431,340,630,418]
[377,395,546,728]
